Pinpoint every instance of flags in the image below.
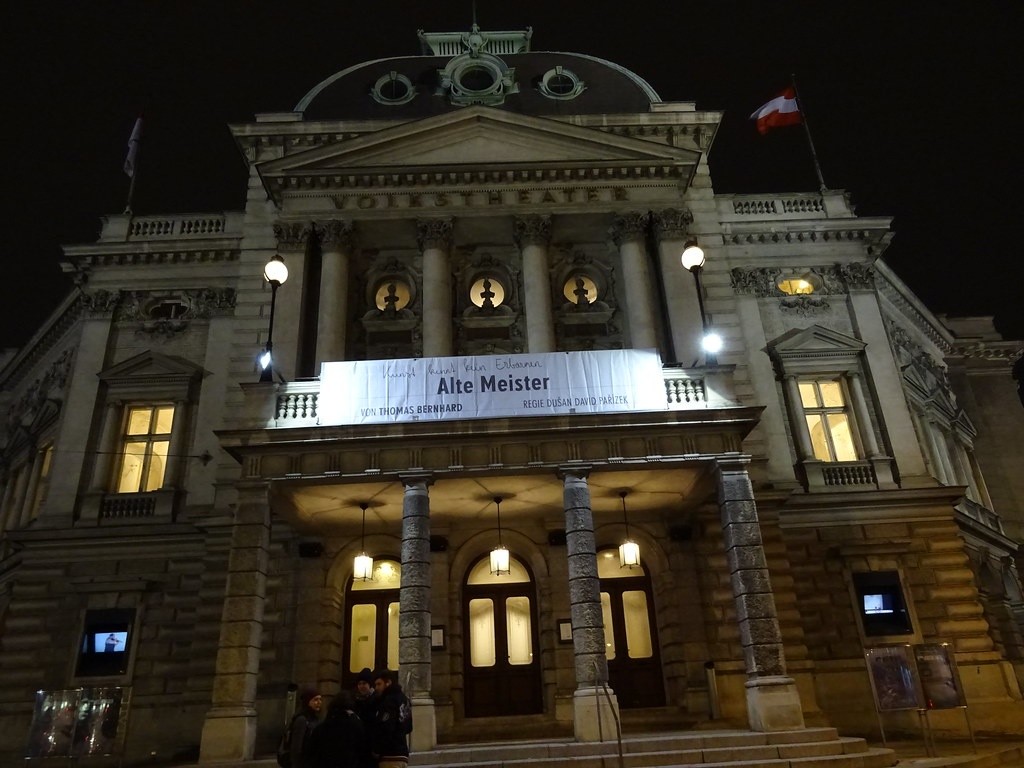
[122,109,144,177]
[748,87,803,136]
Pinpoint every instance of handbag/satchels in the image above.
[277,713,310,768]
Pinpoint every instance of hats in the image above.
[300,688,322,704]
[356,668,372,685]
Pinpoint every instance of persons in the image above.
[288,668,413,768]
[105,634,119,652]
